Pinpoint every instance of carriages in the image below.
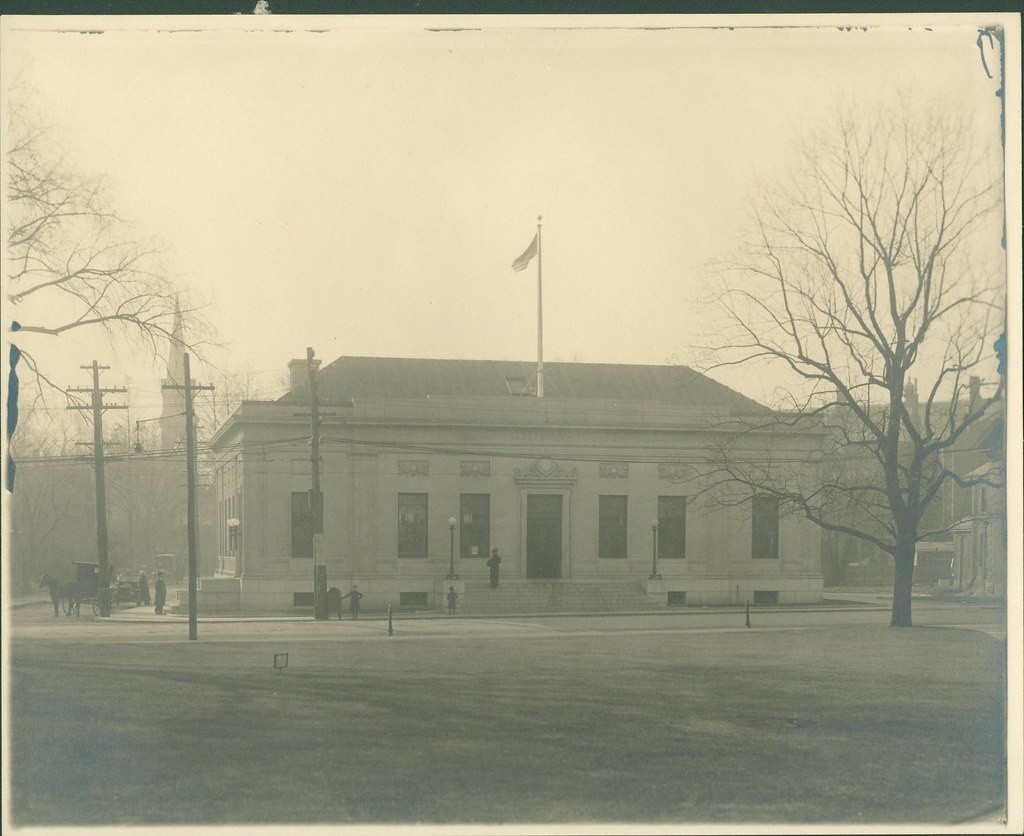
[113,564,145,609]
[151,552,185,586]
[36,559,114,618]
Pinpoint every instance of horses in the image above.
[39,574,82,617]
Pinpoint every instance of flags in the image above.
[510,232,538,273]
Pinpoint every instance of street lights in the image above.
[444,516,460,580]
[646,517,662,581]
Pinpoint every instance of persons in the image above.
[152,572,167,614]
[342,585,364,621]
[486,548,501,586]
[446,586,458,614]
[117,564,152,605]
